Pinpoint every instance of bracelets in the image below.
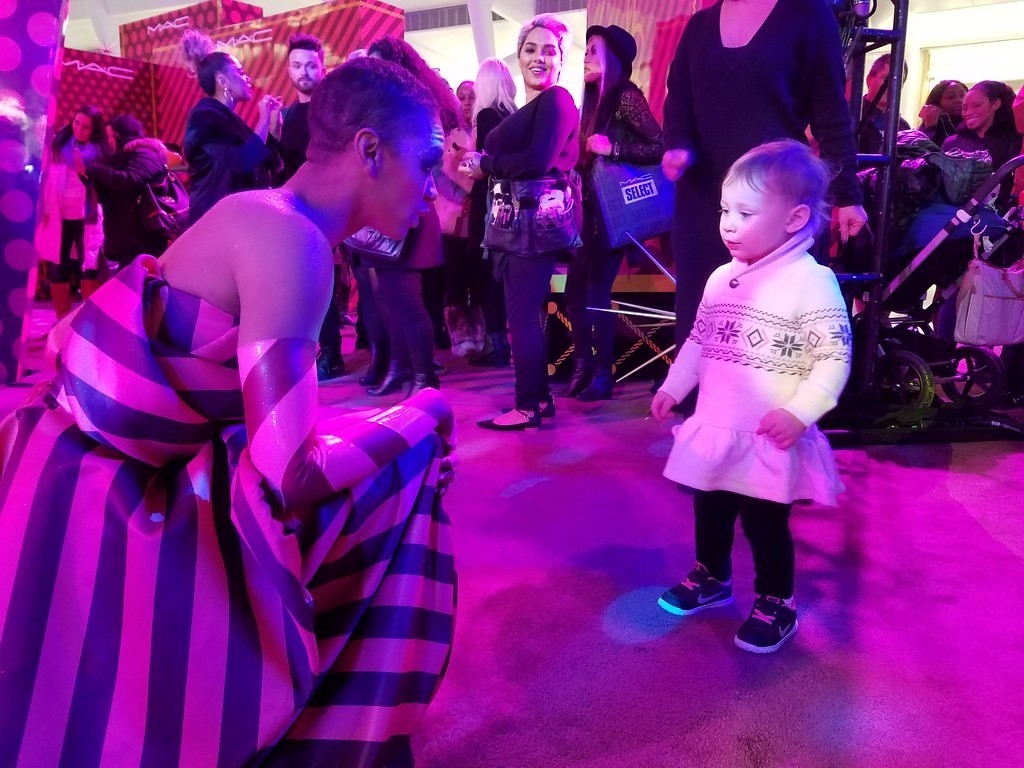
[613,142,621,162]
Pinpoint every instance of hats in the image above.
[586,23,637,74]
[111,116,142,140]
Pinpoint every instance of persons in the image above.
[447,78,499,359]
[859,51,912,145]
[456,11,583,432]
[354,34,443,400]
[86,114,170,274]
[35,105,111,330]
[6,54,459,768]
[166,30,287,234]
[270,34,349,385]
[941,79,1024,206]
[659,1,867,457]
[553,22,669,403]
[465,56,518,365]
[640,138,854,656]
[913,77,969,153]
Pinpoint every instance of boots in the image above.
[563,359,589,398]
[316,338,344,382]
[81,280,97,304]
[404,371,439,401]
[30,281,73,345]
[469,334,503,367]
[575,364,614,401]
[359,341,386,388]
[500,329,510,367]
[369,360,412,397]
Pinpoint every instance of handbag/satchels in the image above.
[139,171,192,238]
[955,261,1024,347]
[481,176,583,255]
[592,159,676,249]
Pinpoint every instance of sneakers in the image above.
[656,563,733,615]
[734,596,798,655]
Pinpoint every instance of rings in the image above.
[262,99,268,104]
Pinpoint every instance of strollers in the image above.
[849,155,1024,428]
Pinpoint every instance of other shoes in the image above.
[500,396,556,416]
[476,408,542,432]
